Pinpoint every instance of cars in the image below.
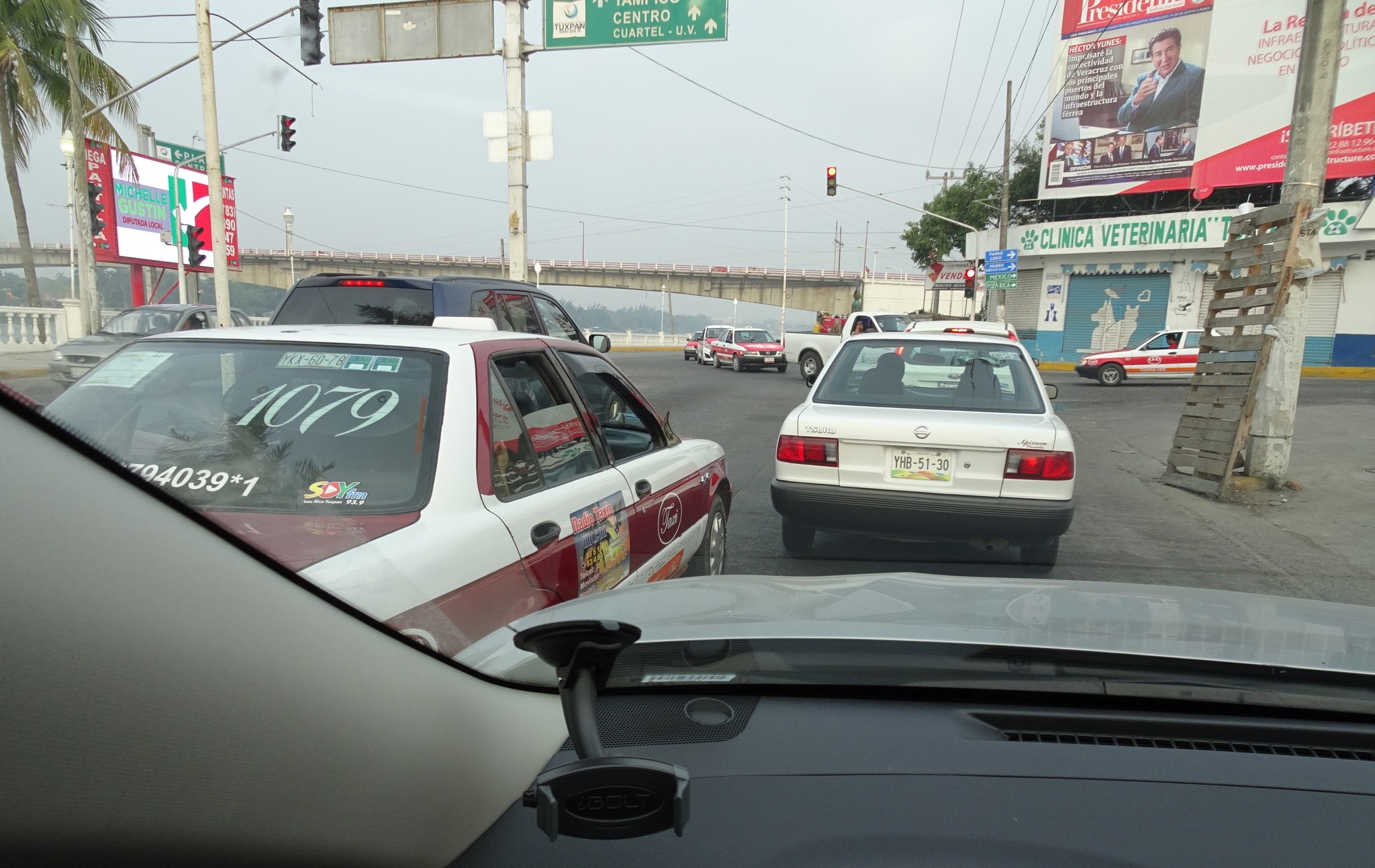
[709,326,788,372]
[1075,329,1233,386]
[894,320,1039,396]
[684,331,703,361]
[769,329,1077,573]
[697,325,735,366]
[38,322,733,659]
[48,304,254,391]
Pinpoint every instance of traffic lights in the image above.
[281,115,297,152]
[827,167,837,196]
[88,182,107,236]
[300,0,325,67]
[187,225,206,267]
[964,269,975,298]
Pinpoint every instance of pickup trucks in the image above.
[783,311,916,381]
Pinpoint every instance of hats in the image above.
[856,320,864,326]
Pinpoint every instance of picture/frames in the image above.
[1129,47,1153,65]
[1130,135,1143,144]
[1160,127,1185,153]
[1096,137,1110,147]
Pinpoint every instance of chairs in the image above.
[859,368,903,400]
[955,369,1002,398]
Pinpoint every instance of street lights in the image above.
[535,262,542,289]
[283,207,296,286]
[60,130,77,298]
[733,298,737,328]
[661,284,666,331]
[857,245,896,311]
[579,221,584,266]
[886,265,917,312]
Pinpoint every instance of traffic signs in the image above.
[154,140,226,175]
[541,0,729,49]
[985,261,1017,274]
[985,272,1017,282]
[985,248,1017,262]
[986,280,1017,290]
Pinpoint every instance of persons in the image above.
[1148,130,1196,160]
[1099,136,1132,165]
[171,313,191,331]
[727,331,744,342]
[1165,334,1178,349]
[851,321,866,335]
[1117,27,1206,132]
[964,358,993,374]
[877,352,906,381]
[491,440,511,497]
[1056,142,1074,168]
[865,266,870,278]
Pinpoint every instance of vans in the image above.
[239,270,627,427]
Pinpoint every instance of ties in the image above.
[1066,158,1069,166]
[1120,147,1123,160]
[1110,154,1112,162]
